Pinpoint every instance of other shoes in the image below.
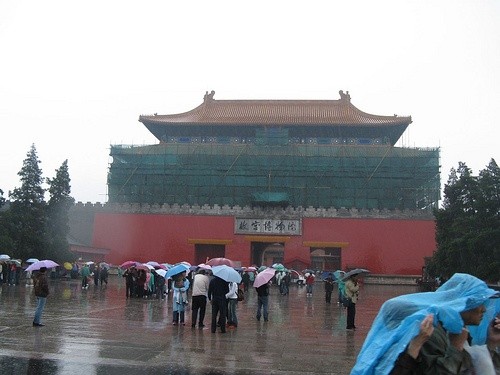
[345,328,353,331]
[173,322,178,325]
[192,325,195,328]
[211,331,216,333]
[33,322,45,327]
[222,331,226,333]
[229,324,235,329]
[180,322,185,325]
[217,323,220,327]
[199,324,204,327]
[225,323,231,326]
[354,327,358,331]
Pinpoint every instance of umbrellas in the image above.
[343,268,371,280]
[99,262,110,270]
[333,269,346,280]
[8,260,21,267]
[121,257,298,288]
[321,271,337,283]
[302,268,314,274]
[24,260,59,272]
[26,258,39,263]
[86,261,94,265]
[0,254,10,261]
[64,261,73,271]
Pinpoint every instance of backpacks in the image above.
[235,288,244,301]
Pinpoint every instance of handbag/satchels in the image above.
[343,297,350,306]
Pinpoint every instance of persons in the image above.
[72,260,111,290]
[32,268,54,327]
[121,269,315,335]
[325,274,334,303]
[0,261,34,288]
[383,273,500,375]
[336,272,345,304]
[343,274,361,332]
